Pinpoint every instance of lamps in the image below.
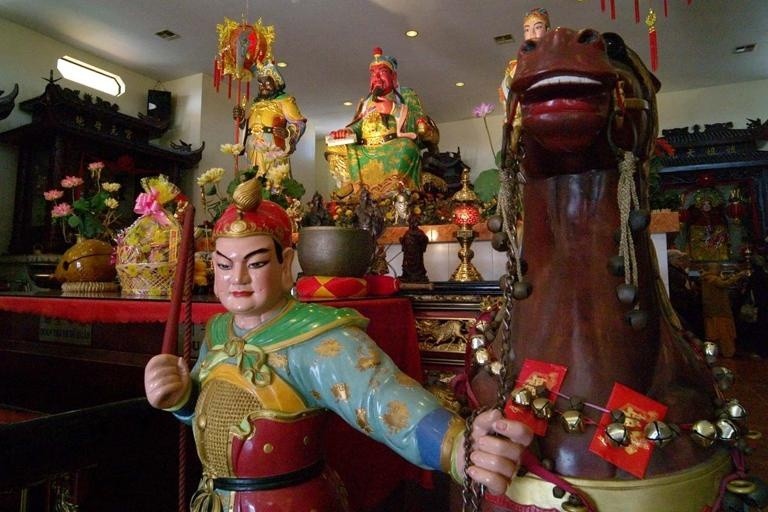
[451,167,484,282]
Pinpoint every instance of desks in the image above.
[0,296,434,512]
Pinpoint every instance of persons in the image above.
[143,163,539,512]
[321,55,440,204]
[498,4,555,146]
[231,62,308,192]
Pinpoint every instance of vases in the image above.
[74,234,86,244]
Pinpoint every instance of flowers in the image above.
[44,161,121,238]
[473,102,502,203]
[196,143,305,223]
